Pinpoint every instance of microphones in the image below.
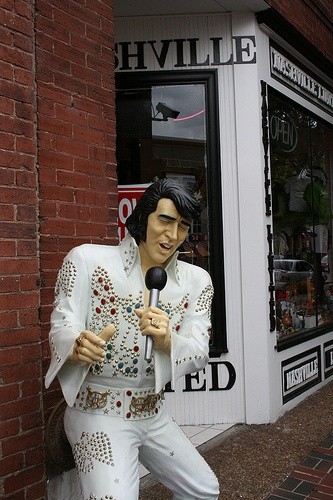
[144,265,168,361]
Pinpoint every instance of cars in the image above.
[273,254,329,298]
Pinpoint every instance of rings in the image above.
[75,346,81,353]
[151,318,159,327]
[77,336,85,344]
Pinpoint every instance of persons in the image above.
[45,178,219,499]
